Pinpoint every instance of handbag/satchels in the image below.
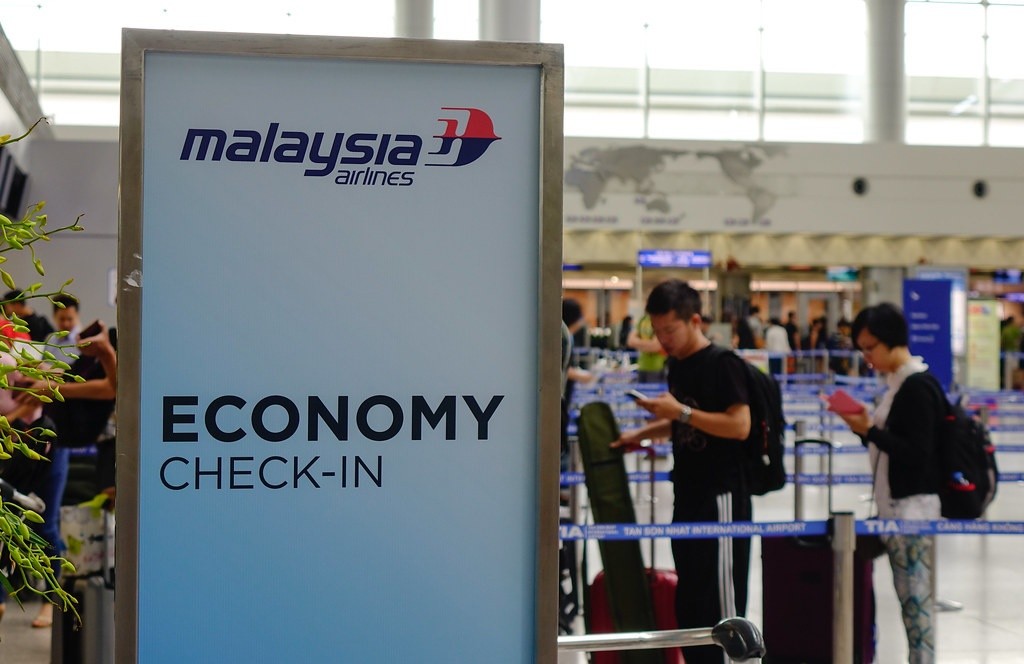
[58,500,116,579]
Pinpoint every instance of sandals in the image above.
[32,614,53,628]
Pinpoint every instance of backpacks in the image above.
[704,345,792,497]
[919,372,998,521]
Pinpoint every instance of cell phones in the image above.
[625,391,642,399]
[78,320,102,340]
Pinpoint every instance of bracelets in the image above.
[736,307,874,378]
[680,407,692,423]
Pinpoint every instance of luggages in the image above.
[58,496,116,664]
[584,442,687,664]
[761,438,877,664]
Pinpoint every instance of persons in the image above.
[560,298,593,507]
[0,290,118,627]
[837,304,949,664]
[609,280,750,664]
[626,312,669,461]
[1001,316,1024,352]
